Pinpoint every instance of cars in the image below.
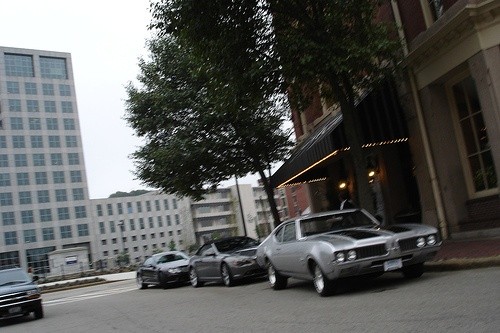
[136,251,190,289]
[187,236,270,287]
[0,268,44,324]
[256,209,443,297]
